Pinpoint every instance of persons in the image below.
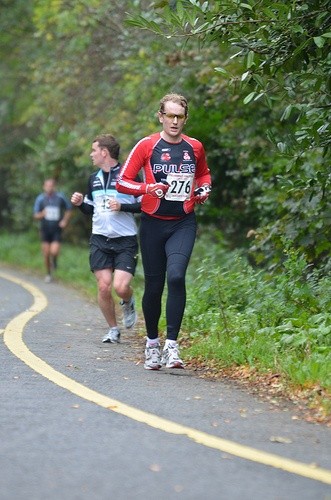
[116,92,211,370]
[70,134,144,343]
[33,177,72,283]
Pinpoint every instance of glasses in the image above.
[162,112,186,119]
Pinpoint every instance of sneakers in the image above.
[144,337,162,369]
[161,339,184,368]
[121,297,135,329]
[102,326,120,344]
